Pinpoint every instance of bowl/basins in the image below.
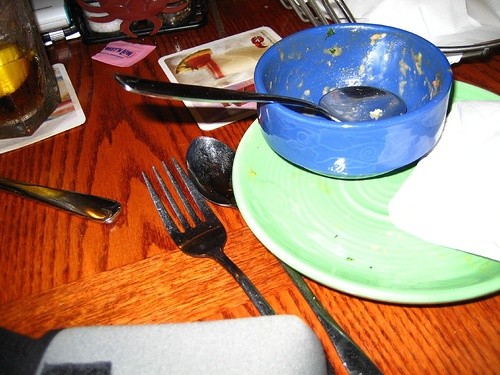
[253,22,454,180]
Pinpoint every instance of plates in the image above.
[77,0,204,44]
[231,78,500,304]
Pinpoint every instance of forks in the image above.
[143,159,275,316]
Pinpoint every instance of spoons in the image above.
[114,73,408,122]
[185,134,384,375]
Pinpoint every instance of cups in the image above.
[0,1,61,140]
